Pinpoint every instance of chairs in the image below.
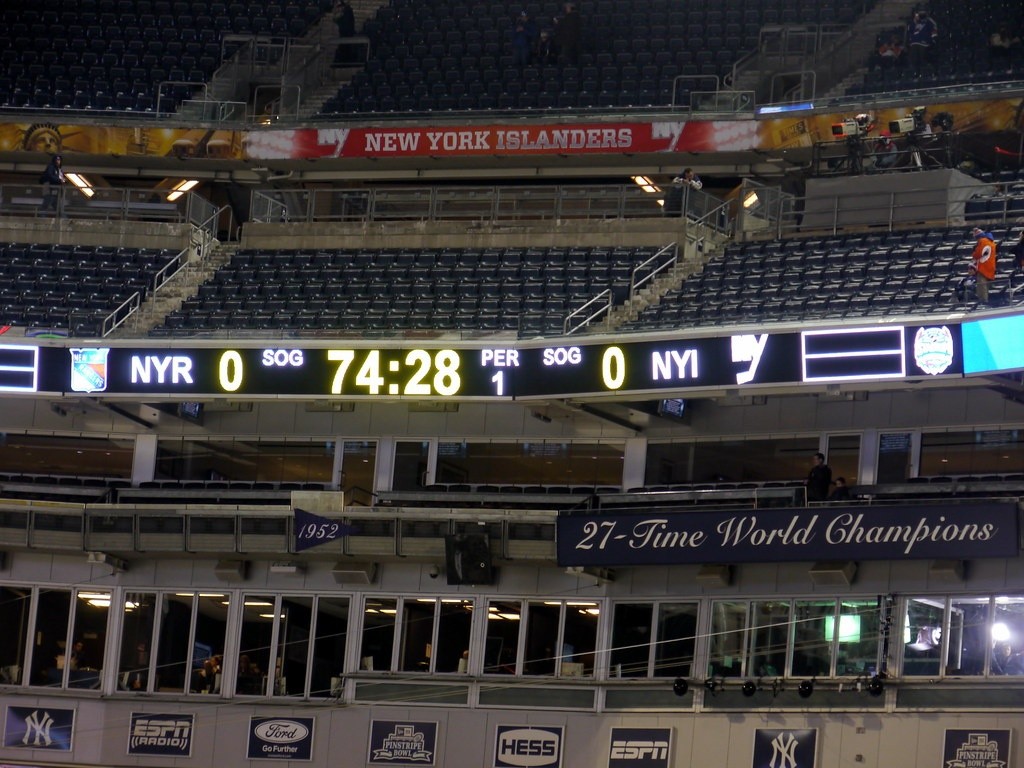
[1,0,1023,511]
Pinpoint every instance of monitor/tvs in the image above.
[178,402,202,421]
[658,399,688,420]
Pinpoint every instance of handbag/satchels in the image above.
[968,259,978,275]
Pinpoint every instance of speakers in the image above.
[696,565,730,588]
[214,561,247,583]
[333,562,378,584]
[809,559,859,587]
[930,558,966,583]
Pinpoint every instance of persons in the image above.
[204,655,260,694]
[39,157,65,211]
[802,453,832,505]
[951,263,976,303]
[832,478,847,499]
[909,8,937,77]
[668,168,702,220]
[55,642,85,667]
[973,228,996,303]
[1015,231,1024,272]
[880,36,902,66]
[333,5,354,52]
[512,3,579,66]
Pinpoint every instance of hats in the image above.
[972,227,983,237]
